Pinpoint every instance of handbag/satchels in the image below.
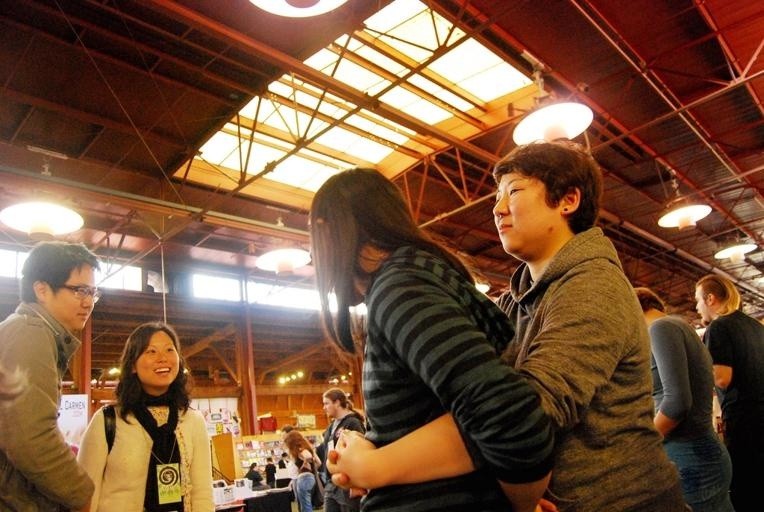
[312,476,326,507]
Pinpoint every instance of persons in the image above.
[307,167,560,512]
[324,125,686,512]
[695,275,763,512]
[240,387,369,512]
[74,318,216,512]
[0,239,102,512]
[632,287,733,512]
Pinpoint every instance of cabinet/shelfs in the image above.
[211,430,328,481]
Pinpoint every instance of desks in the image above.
[215,488,293,512]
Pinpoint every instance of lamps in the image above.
[328,372,353,388]
[512,49,594,146]
[658,168,713,231]
[0,145,86,240]
[248,0,349,18]
[711,234,758,264]
[256,217,310,275]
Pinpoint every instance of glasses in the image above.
[47,283,103,304]
